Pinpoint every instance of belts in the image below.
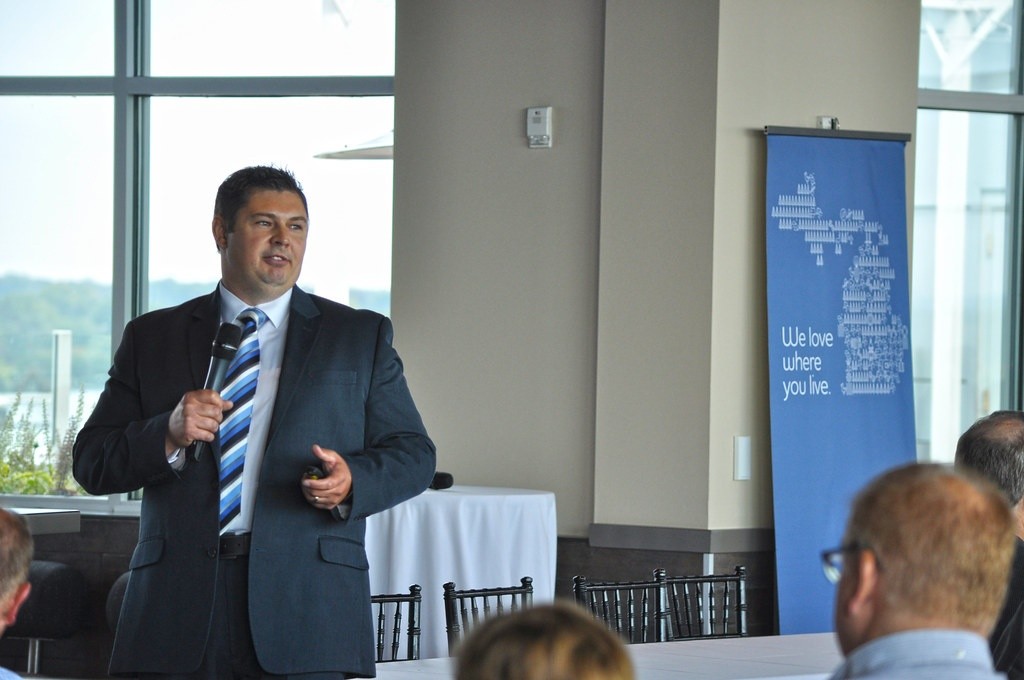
[219,533,251,559]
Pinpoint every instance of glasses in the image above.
[822,543,882,585]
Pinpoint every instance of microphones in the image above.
[430,472,453,492]
[185,323,241,463]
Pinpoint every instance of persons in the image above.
[820,410,1024,680]
[73,165,437,680]
[0,509,35,680]
[453,598,637,680]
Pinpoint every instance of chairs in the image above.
[573,572,668,643]
[371,583,422,663]
[2,560,92,680]
[106,572,131,637]
[443,577,534,657]
[654,565,749,639]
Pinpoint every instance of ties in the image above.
[219,307,269,536]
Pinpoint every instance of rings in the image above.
[312,496,318,504]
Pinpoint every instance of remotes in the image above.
[305,467,324,480]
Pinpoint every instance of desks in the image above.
[365,486,559,658]
[377,630,843,680]
[5,507,81,536]
